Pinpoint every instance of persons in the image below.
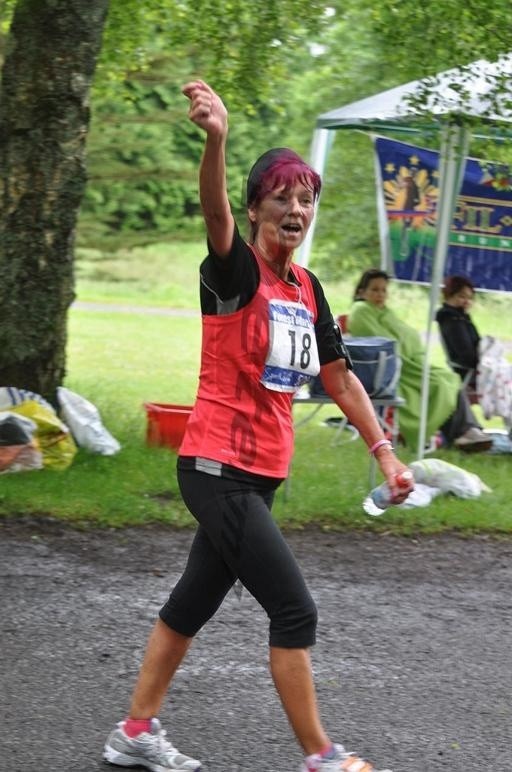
[434,273,483,394]
[100,76,416,771]
[345,265,495,456]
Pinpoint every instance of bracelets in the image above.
[368,438,393,459]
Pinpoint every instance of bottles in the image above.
[362,470,413,516]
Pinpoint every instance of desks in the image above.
[283,384,406,508]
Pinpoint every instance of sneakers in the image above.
[293,742,392,772]
[101,715,204,771]
[455,435,494,452]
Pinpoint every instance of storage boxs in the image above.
[142,400,196,449]
